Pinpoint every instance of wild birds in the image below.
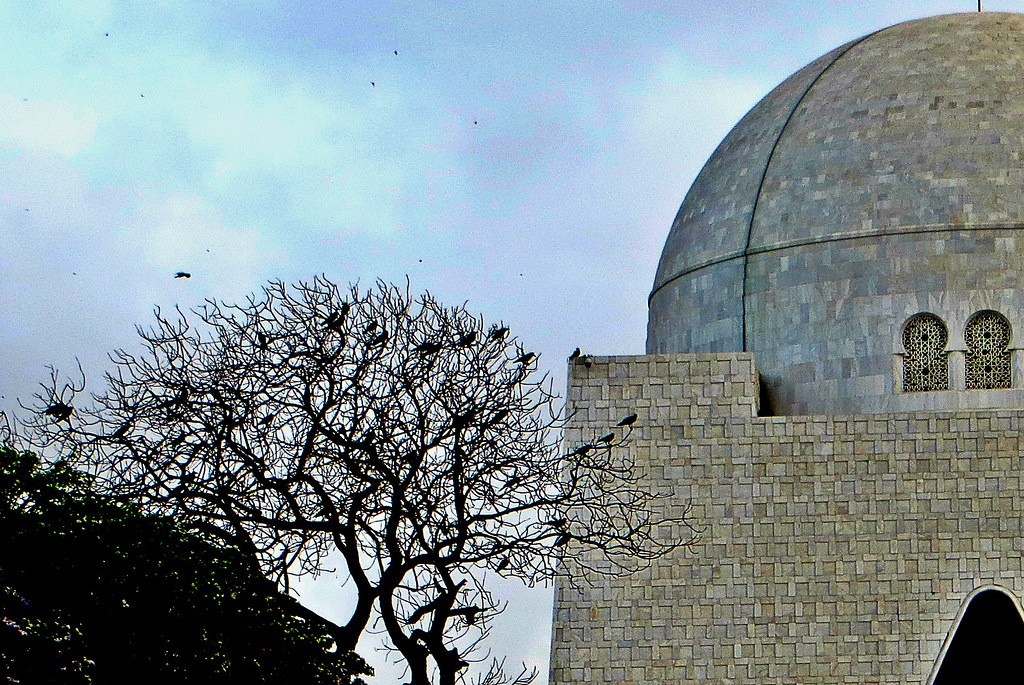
[370,81,375,87]
[519,273,523,276]
[24,98,29,101]
[474,120,478,125]
[40,303,638,573]
[419,259,423,262]
[206,249,210,252]
[105,33,109,36]
[26,209,29,211]
[72,272,76,275]
[394,50,398,54]
[140,93,144,97]
[173,271,192,279]
